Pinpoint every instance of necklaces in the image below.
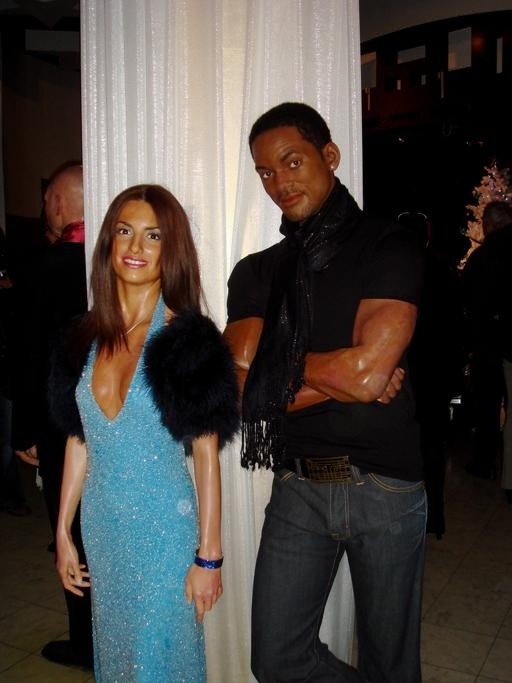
[120,306,156,339]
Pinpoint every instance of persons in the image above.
[394,209,462,549]
[461,198,512,505]
[49,184,242,682]
[218,99,433,683]
[8,162,88,672]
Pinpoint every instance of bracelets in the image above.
[193,548,224,569]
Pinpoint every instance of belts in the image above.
[280,457,379,483]
